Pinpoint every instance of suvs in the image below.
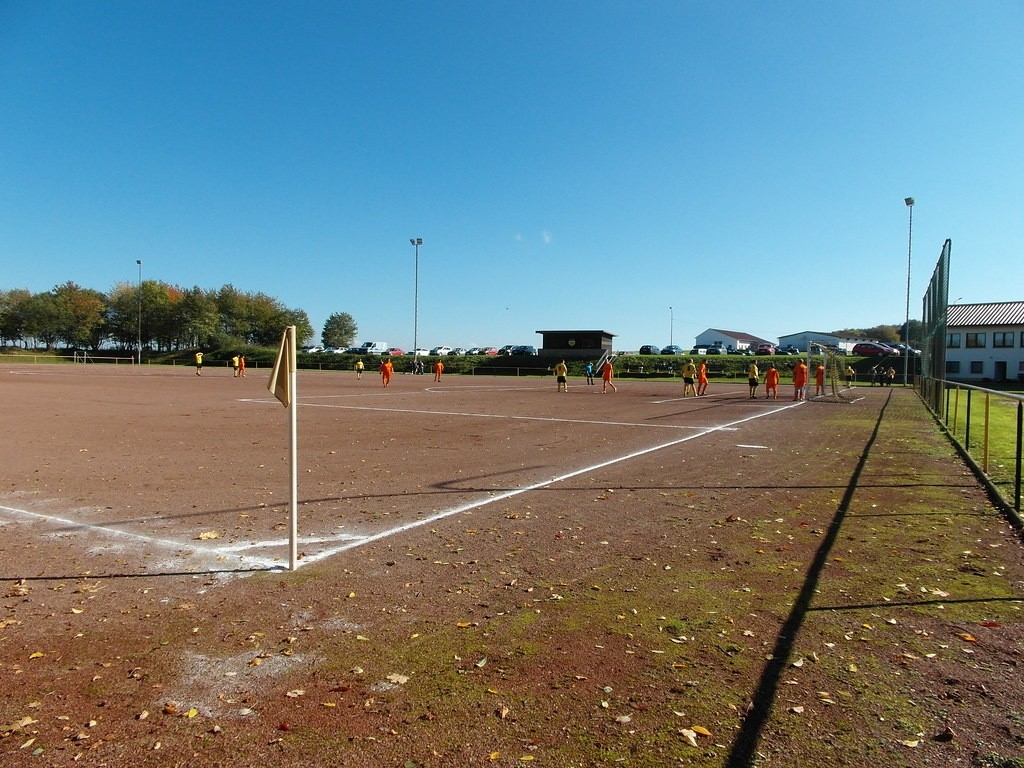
[478,347,498,356]
[328,347,348,354]
[302,346,324,354]
[430,346,453,356]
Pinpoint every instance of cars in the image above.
[317,347,338,353]
[853,342,921,357]
[640,345,661,355]
[661,345,682,355]
[448,348,469,356]
[408,348,430,356]
[755,343,798,355]
[498,345,537,356]
[381,348,405,356]
[465,348,479,355]
[690,341,755,356]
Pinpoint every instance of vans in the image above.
[343,342,387,355]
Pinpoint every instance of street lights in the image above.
[137,260,141,364]
[670,307,673,345]
[905,197,914,388]
[410,238,422,357]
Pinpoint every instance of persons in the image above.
[238,355,246,377]
[586,361,595,385]
[845,366,854,388]
[356,358,364,380]
[887,366,896,387]
[793,359,807,401]
[195,352,203,376]
[870,367,877,386]
[697,359,708,396]
[681,358,698,397]
[816,362,824,395]
[434,359,443,382]
[878,367,886,387]
[379,358,393,387]
[601,360,617,393]
[555,359,568,392]
[409,361,424,375]
[749,359,759,399]
[232,354,240,377]
[763,364,780,399]
